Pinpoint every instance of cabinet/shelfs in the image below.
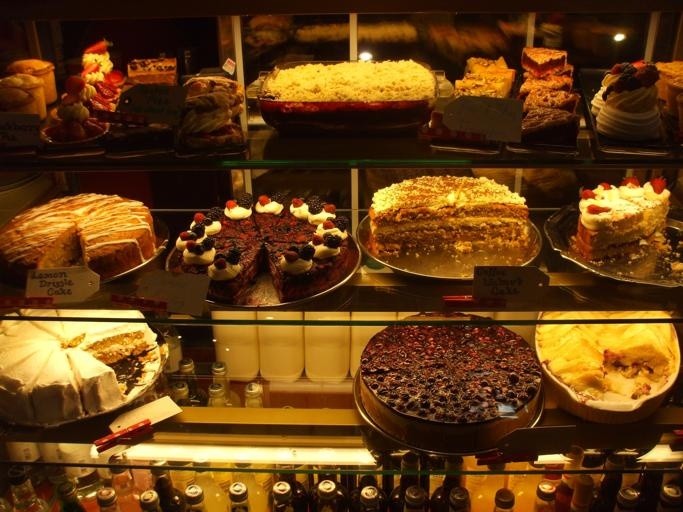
[0,1,683,512]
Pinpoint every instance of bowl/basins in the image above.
[246,60,455,135]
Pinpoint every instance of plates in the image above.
[164,231,360,309]
[0,322,169,430]
[351,364,546,461]
[0,212,171,288]
[542,202,682,289]
[355,207,542,282]
[428,66,675,160]
[35,108,249,164]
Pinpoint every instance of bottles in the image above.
[164,356,266,406]
[0,427,683,512]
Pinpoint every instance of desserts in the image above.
[591,60,682,152]
[454,46,581,152]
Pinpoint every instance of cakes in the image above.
[168,190,359,306]
[0,39,248,164]
[259,46,436,152]
[358,310,544,430]
[0,190,158,288]
[0,305,165,428]
[573,176,673,266]
[366,173,531,257]
